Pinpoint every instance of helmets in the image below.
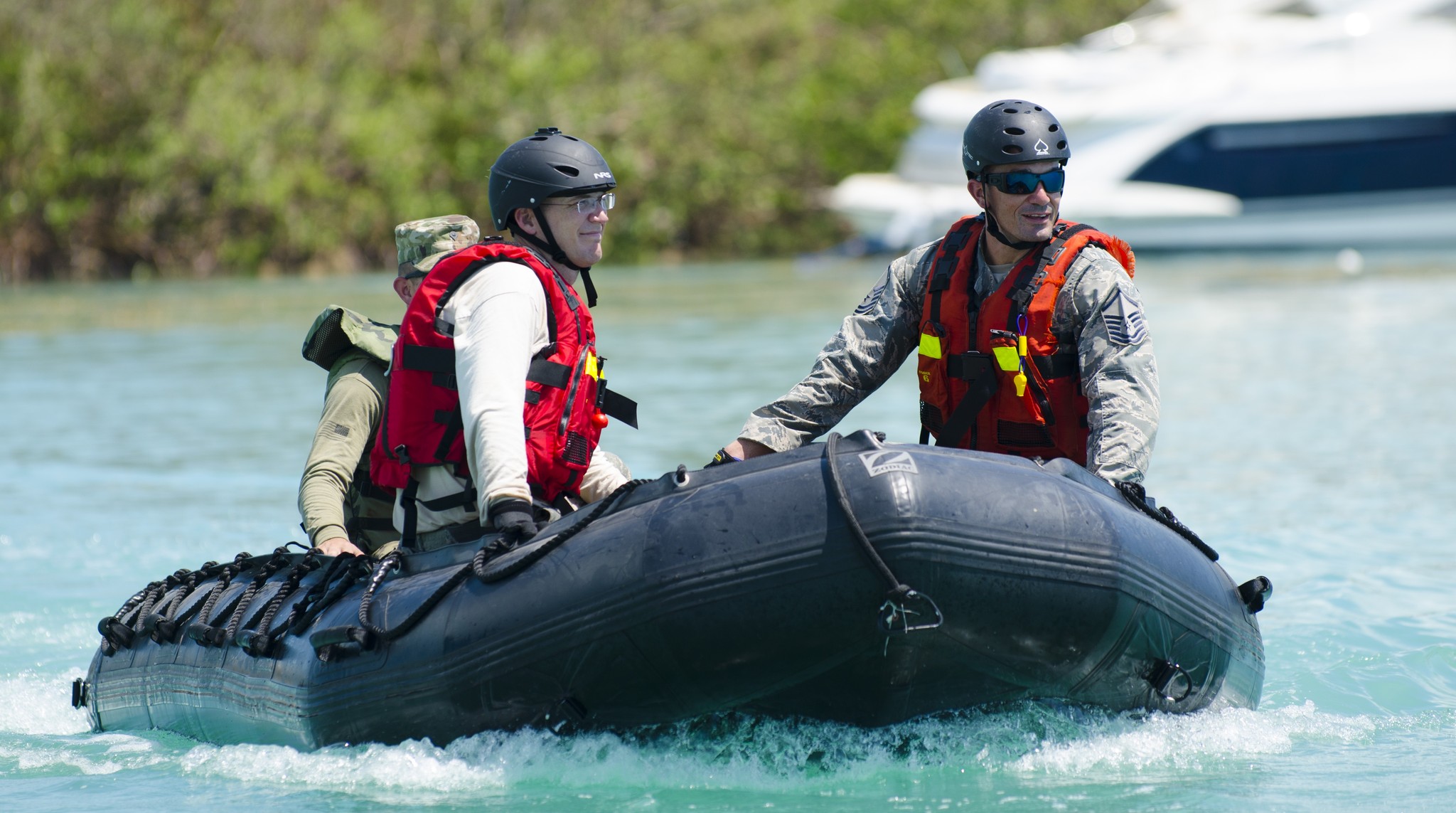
[488,126,616,234]
[962,100,1071,175]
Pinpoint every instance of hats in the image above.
[395,214,480,272]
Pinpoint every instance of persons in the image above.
[297,129,637,557]
[704,100,1161,487]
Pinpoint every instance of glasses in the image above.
[540,193,615,214]
[976,170,1065,195]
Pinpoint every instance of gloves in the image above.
[492,502,551,537]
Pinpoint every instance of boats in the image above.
[818,0,1456,257]
[66,424,1272,769]
